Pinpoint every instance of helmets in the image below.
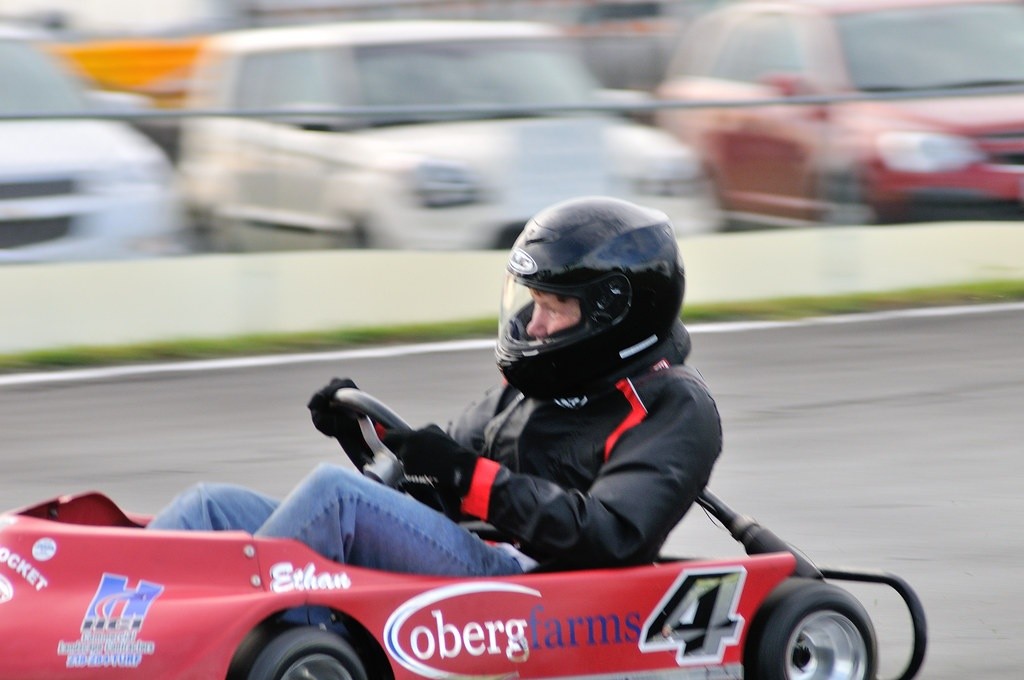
[494,197,691,401]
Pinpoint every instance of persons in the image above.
[147,195,723,576]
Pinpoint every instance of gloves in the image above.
[382,423,474,494]
[308,377,359,437]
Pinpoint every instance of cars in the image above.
[655,0,1024,226]
[169,19,731,260]
[1,22,196,267]
